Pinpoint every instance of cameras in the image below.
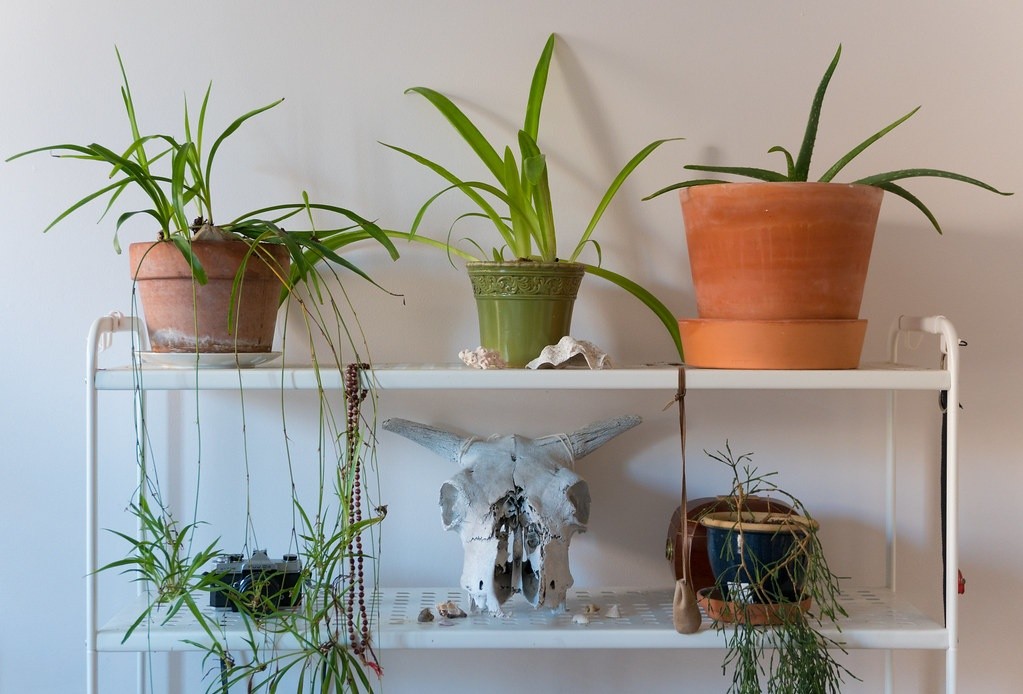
[211,550,302,617]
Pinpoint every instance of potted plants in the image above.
[6,43,405,694]
[379,34,685,370]
[689,437,865,693]
[638,41,1016,370]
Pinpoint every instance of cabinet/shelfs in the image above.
[83,312,963,694]
